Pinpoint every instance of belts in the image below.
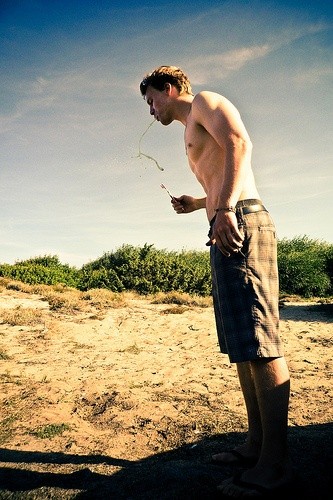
[209,204,268,230]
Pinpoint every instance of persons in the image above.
[138,65,292,499]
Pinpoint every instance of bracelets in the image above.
[217,207,237,213]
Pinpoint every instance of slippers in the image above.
[210,450,298,500]
